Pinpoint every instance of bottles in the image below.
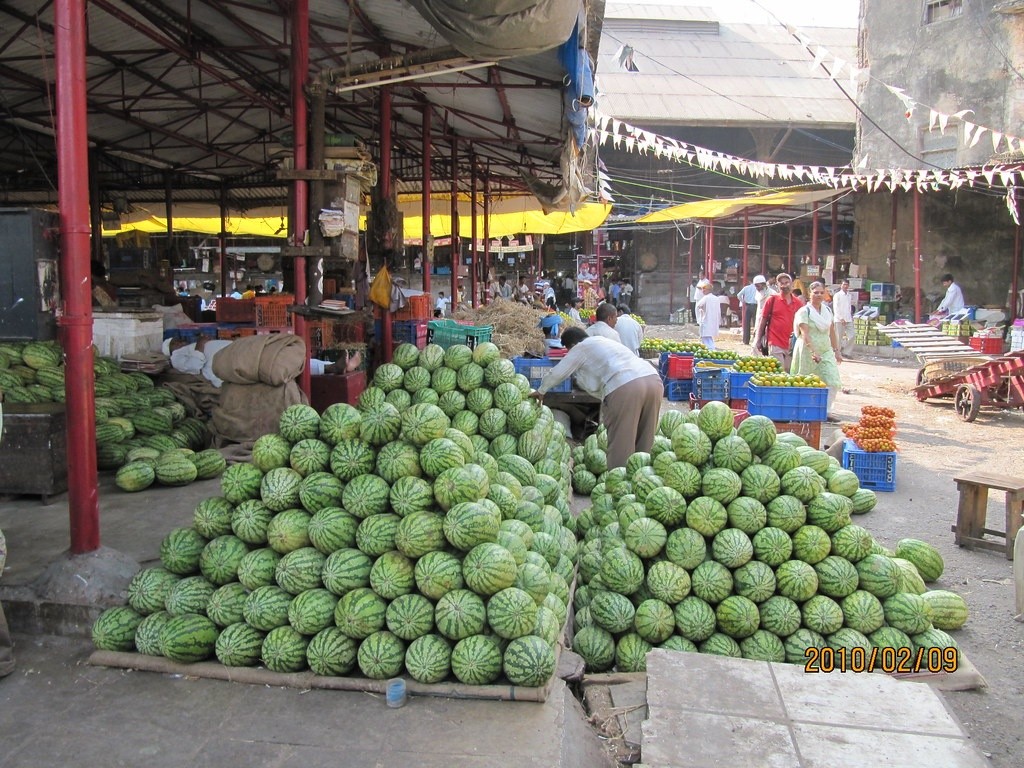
[761,333,768,355]
[346,295,354,311]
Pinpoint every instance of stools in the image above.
[0,401,69,506]
[950,471,1024,560]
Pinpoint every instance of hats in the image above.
[777,272,792,283]
[753,274,767,284]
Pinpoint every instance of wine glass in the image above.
[788,335,794,356]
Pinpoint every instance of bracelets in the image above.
[834,348,840,352]
[809,350,816,355]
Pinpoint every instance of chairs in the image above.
[978,311,1005,327]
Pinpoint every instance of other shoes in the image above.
[841,353,853,360]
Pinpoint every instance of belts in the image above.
[746,303,756,305]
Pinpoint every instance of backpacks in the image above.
[787,307,831,357]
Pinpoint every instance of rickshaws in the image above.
[910,349,1024,423]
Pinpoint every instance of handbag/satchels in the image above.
[761,336,769,357]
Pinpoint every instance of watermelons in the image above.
[90,402,581,687]
[569,402,966,676]
[351,342,576,533]
[0,339,225,490]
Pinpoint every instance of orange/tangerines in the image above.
[639,337,739,360]
[731,355,827,388]
[556,309,595,322]
[630,314,646,325]
[841,406,897,453]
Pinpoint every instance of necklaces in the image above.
[529,327,666,470]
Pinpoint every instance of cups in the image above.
[386,678,406,708]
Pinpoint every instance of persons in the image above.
[434,272,642,357]
[932,274,965,315]
[790,282,844,423]
[176,284,278,309]
[579,262,597,279]
[752,273,804,372]
[687,270,854,359]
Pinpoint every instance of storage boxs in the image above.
[436,267,449,275]
[773,421,820,449]
[375,292,431,321]
[731,409,748,429]
[692,367,730,399]
[729,371,754,400]
[374,318,424,350]
[667,353,695,379]
[668,378,693,401]
[730,399,747,409]
[799,254,902,347]
[746,380,829,422]
[693,357,736,368]
[939,307,1024,354]
[660,376,669,397]
[659,353,694,375]
[842,440,896,493]
[426,319,493,352]
[689,392,729,410]
[514,357,570,393]
[308,371,368,408]
[93,296,335,358]
